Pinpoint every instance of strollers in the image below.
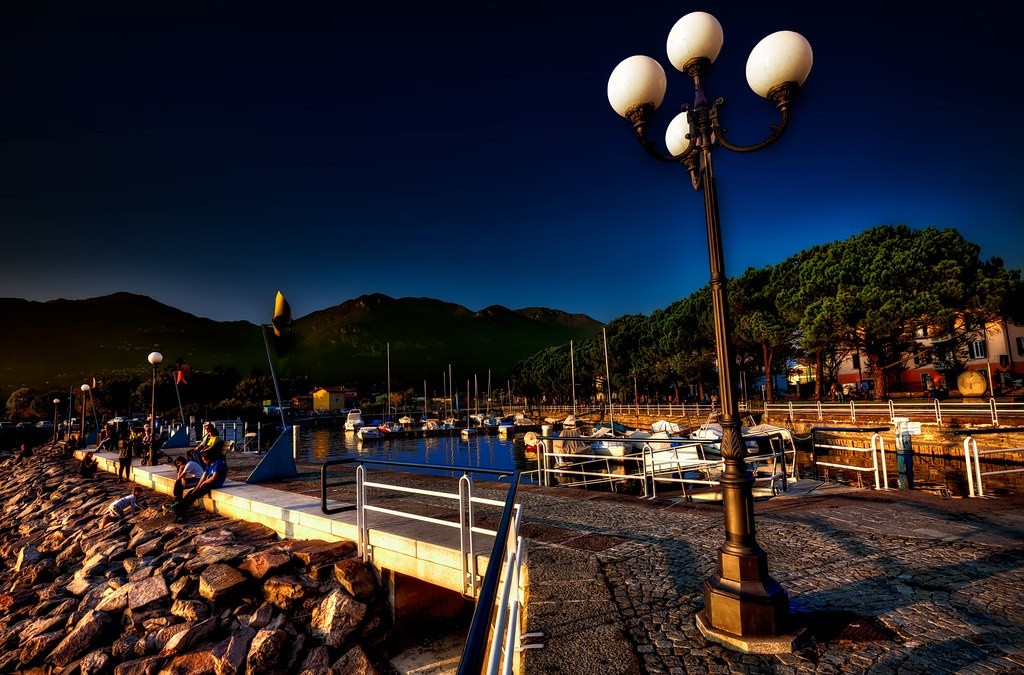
[138,430,173,465]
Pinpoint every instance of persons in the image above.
[187,424,227,471]
[166,457,204,507]
[63,435,77,456]
[14,444,33,465]
[169,453,227,516]
[79,452,98,477]
[831,382,843,403]
[68,435,85,457]
[552,398,555,405]
[116,440,132,484]
[581,399,590,406]
[93,423,164,459]
[710,393,719,404]
[686,394,697,403]
[98,487,146,530]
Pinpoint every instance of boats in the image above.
[202,418,266,455]
[342,325,798,485]
[107,415,137,427]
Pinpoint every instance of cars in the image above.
[16,422,34,429]
[0,421,16,430]
[58,417,89,430]
[262,406,349,416]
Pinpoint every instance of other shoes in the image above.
[124,479,130,482]
[116,479,122,484]
[173,498,183,504]
[161,502,180,516]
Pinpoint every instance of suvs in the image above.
[36,420,52,428]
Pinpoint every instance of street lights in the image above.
[147,351,163,466]
[604,10,815,653]
[79,383,90,448]
[53,399,61,444]
